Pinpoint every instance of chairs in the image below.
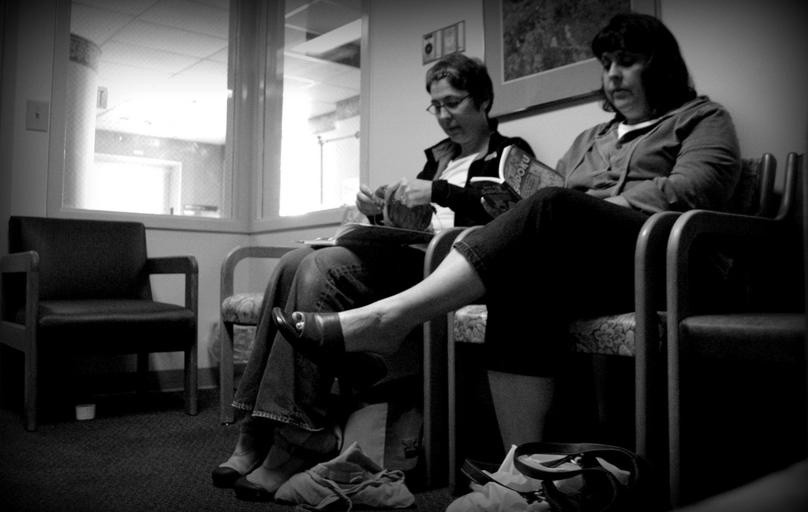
[443,153,776,500]
[663,156,806,511]
[222,225,464,490]
[0,214,198,430]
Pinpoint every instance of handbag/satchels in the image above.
[446,441,659,512]
[274,441,415,512]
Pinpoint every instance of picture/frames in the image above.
[482,0,655,119]
[421,19,465,64]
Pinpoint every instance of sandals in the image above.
[426,90,475,115]
[272,305,395,370]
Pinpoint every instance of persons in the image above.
[271,11,741,511]
[209,48,538,502]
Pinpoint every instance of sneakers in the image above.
[212,416,299,501]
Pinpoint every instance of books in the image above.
[469,144,568,214]
[303,222,438,250]
